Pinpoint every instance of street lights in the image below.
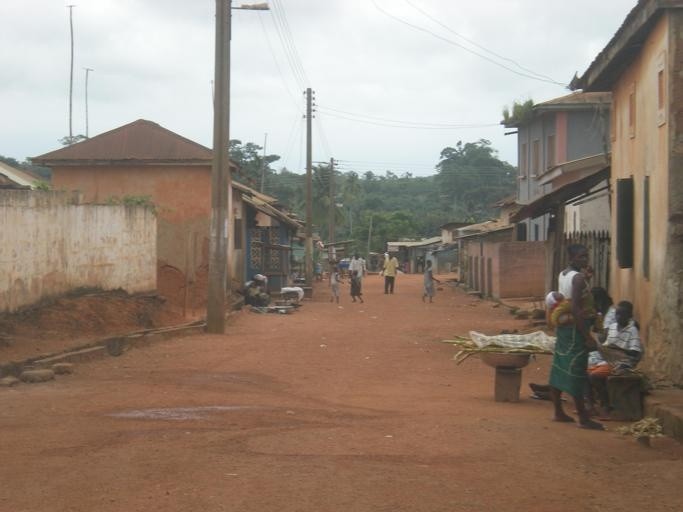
[327,202,343,261]
[201,0,273,334]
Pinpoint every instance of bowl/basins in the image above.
[478,350,530,370]
[274,306,293,314]
[275,301,289,306]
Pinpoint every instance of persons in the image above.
[404,260,410,274]
[381,253,399,294]
[329,267,344,304]
[377,254,385,275]
[418,257,424,272]
[544,241,642,429]
[348,254,366,302]
[289,261,323,285]
[422,261,440,302]
[244,274,271,307]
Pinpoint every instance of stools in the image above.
[491,366,522,403]
[604,371,644,422]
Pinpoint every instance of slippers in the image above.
[576,422,605,430]
[551,414,574,421]
